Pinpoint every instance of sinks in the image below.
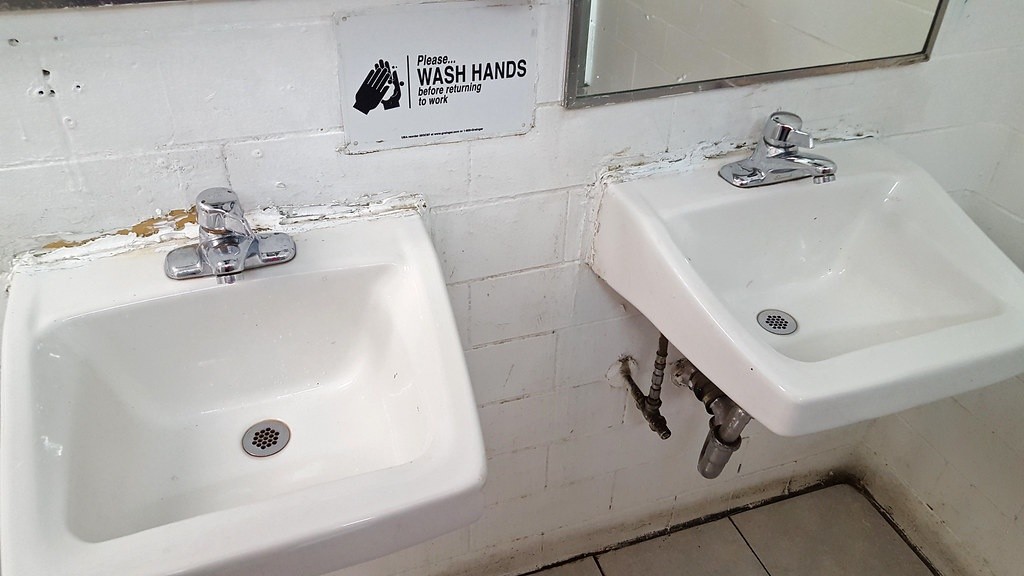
[0,209,488,576]
[588,137,1024,437]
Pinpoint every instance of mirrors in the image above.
[563,0,952,109]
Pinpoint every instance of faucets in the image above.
[164,188,296,284]
[719,112,837,187]
